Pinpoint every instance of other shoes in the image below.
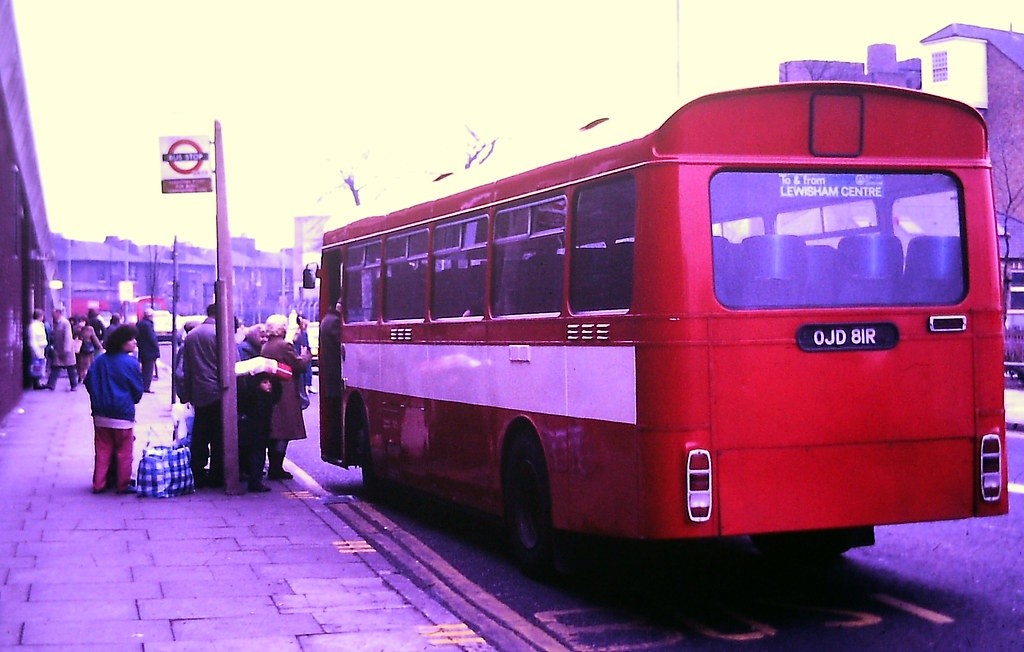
[71,386,78,391]
[247,484,272,492]
[78,377,81,383]
[239,474,251,482]
[83,380,85,384]
[33,386,46,390]
[144,391,155,393]
[42,383,55,391]
[268,468,294,480]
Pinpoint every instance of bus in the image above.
[303,81,1009,577]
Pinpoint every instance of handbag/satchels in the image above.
[82,339,96,354]
[171,402,195,446]
[72,334,82,354]
[30,359,47,378]
[136,427,196,498]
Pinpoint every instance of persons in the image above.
[83,323,146,493]
[319,299,343,463]
[172,304,315,491]
[27,298,161,393]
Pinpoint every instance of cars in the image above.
[153,310,183,346]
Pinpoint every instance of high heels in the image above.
[308,390,318,395]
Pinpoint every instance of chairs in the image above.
[367,233,962,320]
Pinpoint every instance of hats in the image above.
[105,324,139,354]
[88,308,100,319]
[112,313,121,319]
[143,308,155,315]
